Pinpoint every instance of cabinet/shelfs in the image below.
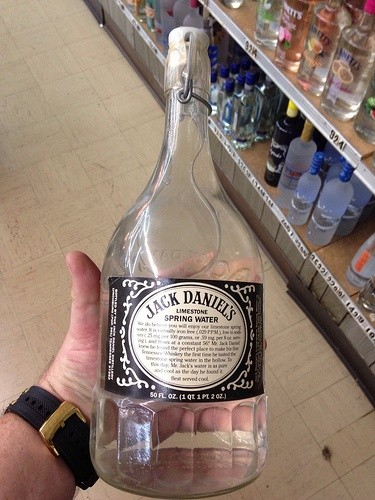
[82,0,375,410]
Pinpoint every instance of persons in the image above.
[0,250,269,500]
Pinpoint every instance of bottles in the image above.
[125,0,375,311]
[88,25,268,500]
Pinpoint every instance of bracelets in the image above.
[5,385,100,490]
